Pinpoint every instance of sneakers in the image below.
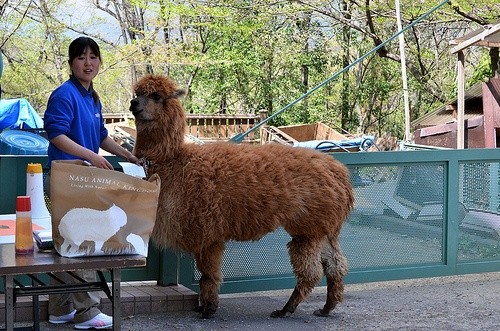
[49,310,76,324]
[74,312,113,329]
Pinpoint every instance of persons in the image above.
[44,37,144,329]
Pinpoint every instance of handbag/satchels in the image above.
[50,159,161,259]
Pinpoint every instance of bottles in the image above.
[15,196,34,255]
[26,161,44,220]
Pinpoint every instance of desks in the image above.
[0,242,147,331]
